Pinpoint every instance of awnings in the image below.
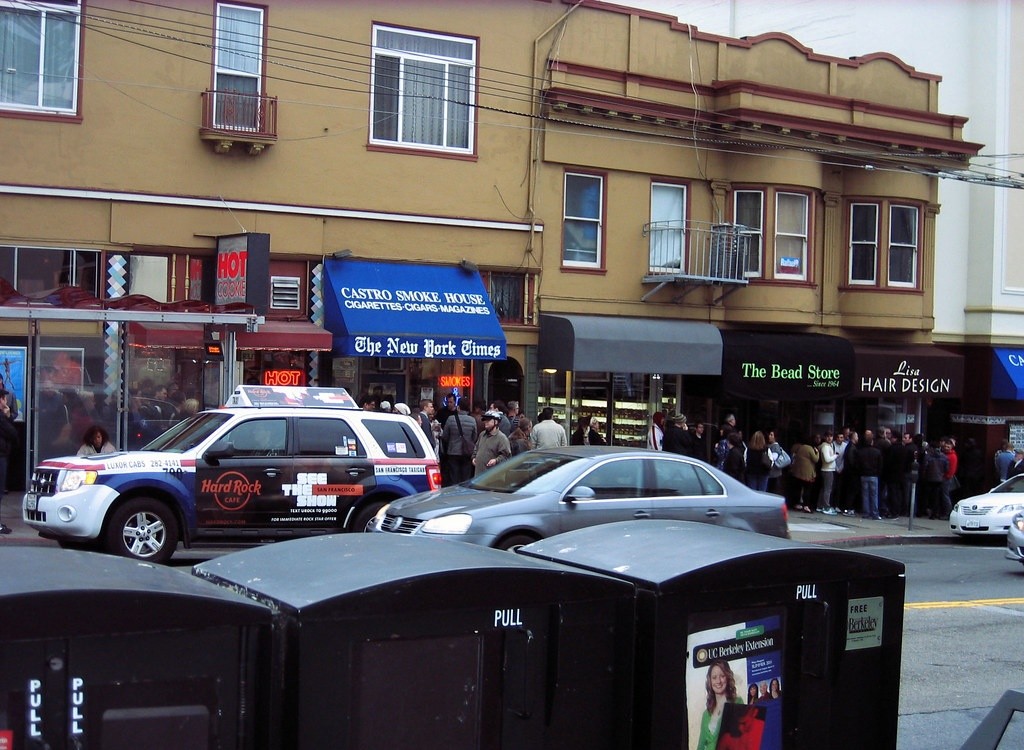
[854,345,965,398]
[717,329,855,393]
[975,346,1024,400]
[537,312,723,375]
[323,259,507,360]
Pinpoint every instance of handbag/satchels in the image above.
[775,448,792,468]
[462,439,474,456]
[946,476,960,491]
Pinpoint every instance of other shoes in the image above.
[861,514,870,518]
[822,508,837,516]
[835,507,841,512]
[873,515,882,520]
[803,507,813,514]
[817,507,823,512]
[843,511,856,517]
[795,504,802,510]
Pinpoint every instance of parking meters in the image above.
[907,459,919,530]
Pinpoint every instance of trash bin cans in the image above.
[193,530,640,750]
[0,544,284,750]
[517,519,906,750]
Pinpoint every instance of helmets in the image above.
[481,410,501,421]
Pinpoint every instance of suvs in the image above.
[21,405,442,564]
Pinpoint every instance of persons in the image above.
[358,394,411,416]
[715,413,782,494]
[0,373,18,535]
[570,413,608,445]
[468,400,512,477]
[647,411,708,463]
[92,388,117,444]
[76,425,117,457]
[959,437,1024,498]
[128,375,186,451]
[180,399,199,419]
[411,399,443,461]
[506,401,568,456]
[791,425,957,520]
[435,393,478,486]
[696,659,781,750]
[38,377,89,462]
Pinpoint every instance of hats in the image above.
[395,403,411,415]
[675,413,687,424]
[1014,449,1024,454]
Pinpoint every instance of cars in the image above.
[950,474,1024,547]
[363,445,792,553]
[1005,511,1024,565]
